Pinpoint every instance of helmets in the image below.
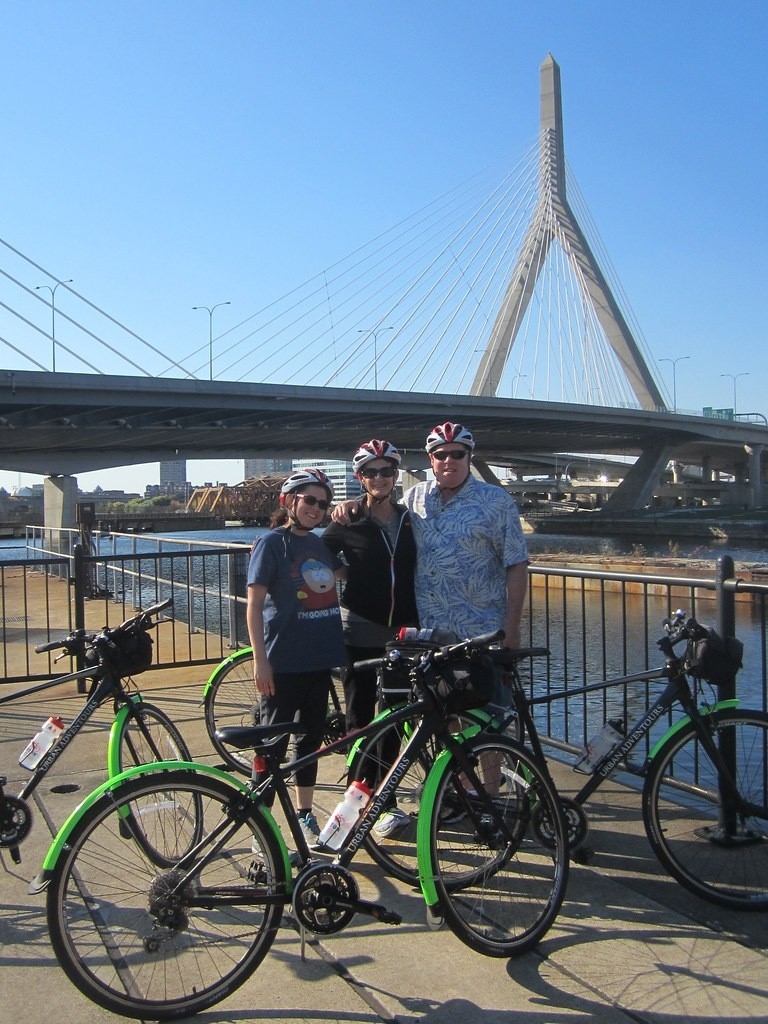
[351,440,400,472]
[280,468,336,502]
[425,421,475,455]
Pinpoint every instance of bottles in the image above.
[319,778,371,849]
[19,716,65,770]
[396,627,434,642]
[575,717,625,774]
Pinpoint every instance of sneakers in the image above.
[480,801,504,840]
[293,814,325,849]
[439,787,483,825]
[253,830,298,865]
[376,808,410,837]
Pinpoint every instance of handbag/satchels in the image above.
[427,655,493,705]
[683,627,744,682]
[81,631,153,680]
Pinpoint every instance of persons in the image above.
[246,469,348,865]
[330,421,530,843]
[250,439,418,833]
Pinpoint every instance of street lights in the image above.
[721,372,749,421]
[474,347,506,397]
[34,279,73,372]
[587,387,600,405]
[511,374,527,399]
[192,302,231,380]
[659,356,690,414]
[358,327,394,391]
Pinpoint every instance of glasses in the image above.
[359,466,397,478]
[430,449,471,460]
[297,493,329,510]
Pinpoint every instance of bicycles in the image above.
[27,630,569,1021]
[0,598,204,870]
[197,638,525,794]
[338,609,768,959]
